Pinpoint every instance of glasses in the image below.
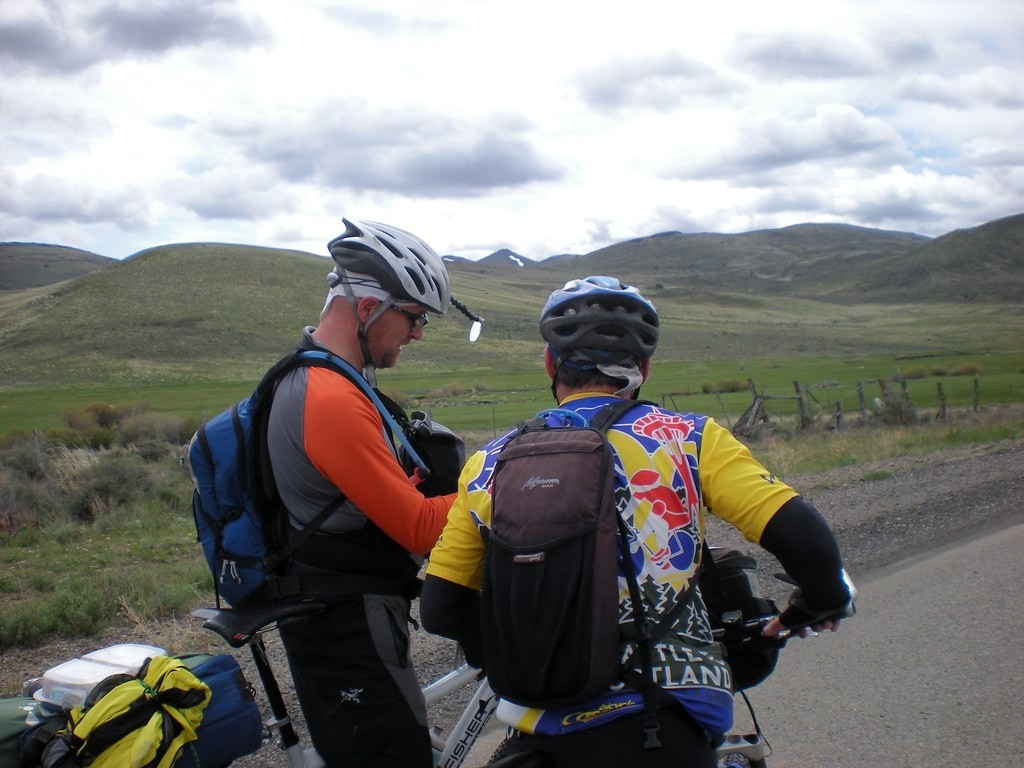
[389,305,428,331]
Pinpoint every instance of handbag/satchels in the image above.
[699,546,785,692]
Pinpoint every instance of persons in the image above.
[255,216,461,768]
[419,274,858,768]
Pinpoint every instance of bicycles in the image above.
[710,600,856,768]
[191,607,529,768]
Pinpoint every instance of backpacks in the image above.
[186,354,383,609]
[479,398,659,714]
[375,388,466,601]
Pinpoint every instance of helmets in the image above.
[325,217,451,314]
[539,276,660,368]
